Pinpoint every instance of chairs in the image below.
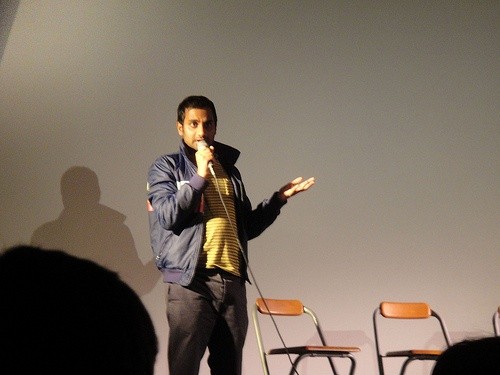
[252,297,360,375]
[372,302,452,375]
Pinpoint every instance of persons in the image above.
[147,95,315,375]
[0,245,159,374]
[430,335,500,375]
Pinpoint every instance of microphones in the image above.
[198,141,215,176]
